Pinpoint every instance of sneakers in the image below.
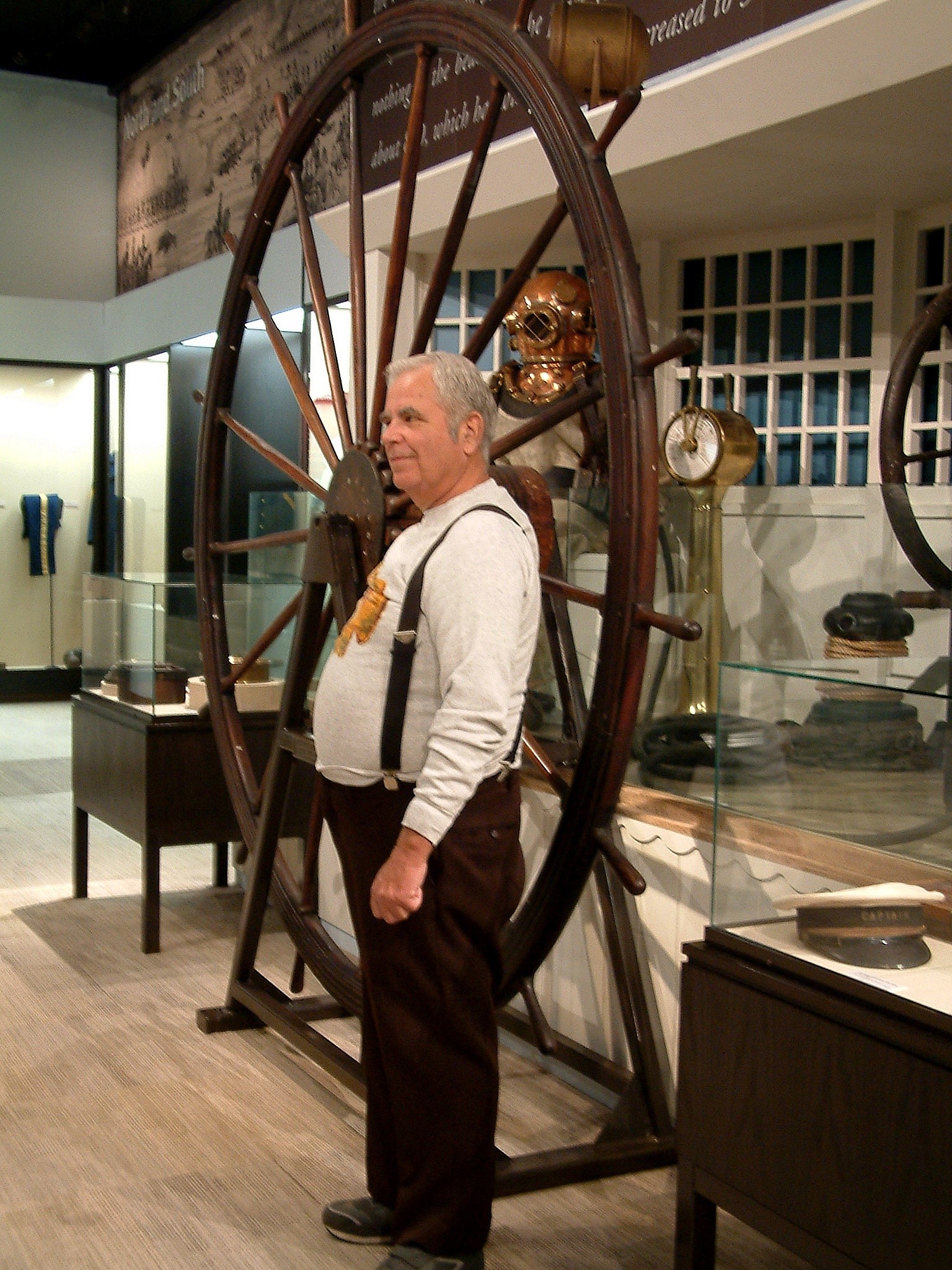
[321,1197,403,1244]
[377,1247,464,1270]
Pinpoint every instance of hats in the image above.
[773,883,945,969]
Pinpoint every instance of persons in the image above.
[313,351,543,1270]
